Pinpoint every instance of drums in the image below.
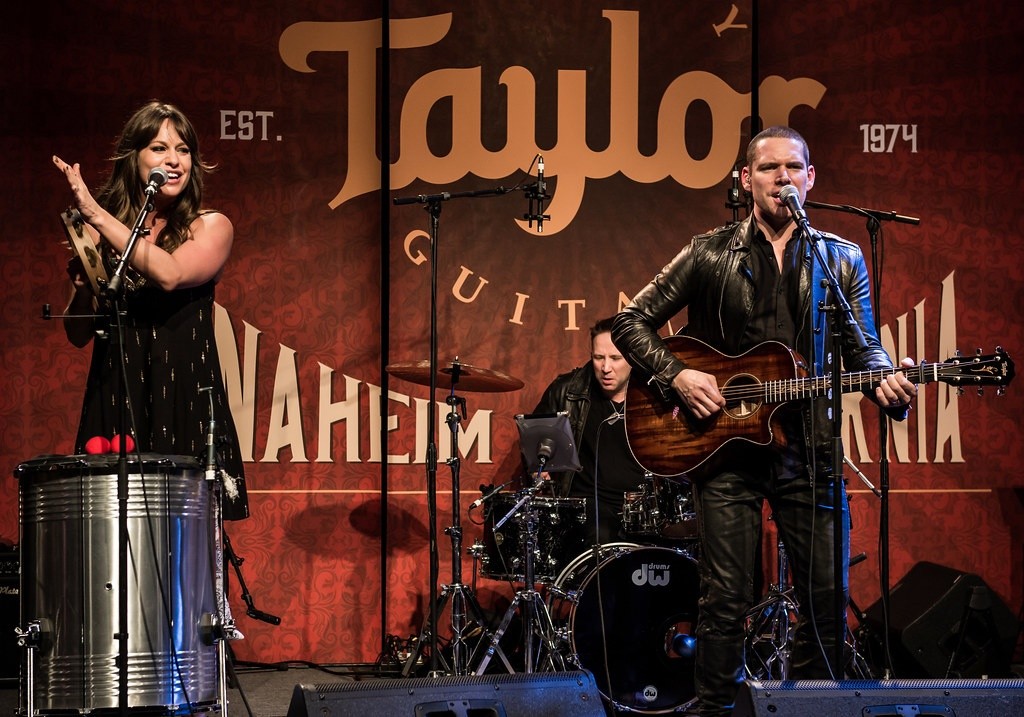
[61,208,109,297]
[14,453,220,717]
[622,490,696,536]
[546,542,708,717]
[481,496,588,583]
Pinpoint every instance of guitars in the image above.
[625,335,1015,476]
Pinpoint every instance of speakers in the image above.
[861,558,1022,679]
[733,678,1024,717]
[288,668,609,717]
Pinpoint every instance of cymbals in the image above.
[386,359,526,393]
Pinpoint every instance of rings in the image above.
[893,399,899,404]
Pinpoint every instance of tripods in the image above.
[401,373,564,675]
[742,535,874,681]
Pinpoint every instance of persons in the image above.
[53,102,251,520]
[611,126,918,717]
[521,317,644,549]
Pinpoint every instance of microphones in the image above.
[730,168,739,225]
[674,633,696,656]
[535,156,545,233]
[467,479,515,509]
[779,185,808,227]
[144,166,169,196]
